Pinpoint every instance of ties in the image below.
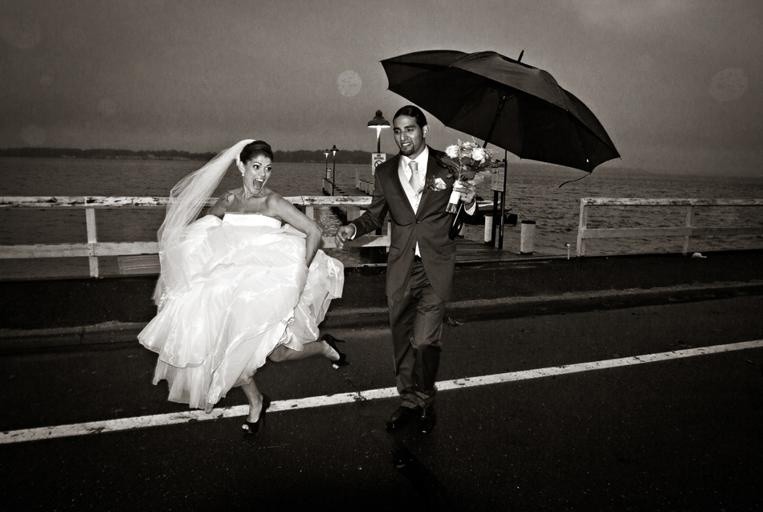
[410,161,420,196]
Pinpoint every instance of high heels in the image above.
[239,392,271,434]
[320,333,346,372]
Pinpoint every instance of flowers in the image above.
[440,135,498,213]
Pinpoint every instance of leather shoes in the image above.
[386,403,438,439]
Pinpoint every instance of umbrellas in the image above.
[378,47,623,240]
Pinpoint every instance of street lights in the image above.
[322,149,329,181]
[366,110,391,155]
[330,145,338,194]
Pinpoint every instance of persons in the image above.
[334,104,482,435]
[136,137,350,435]
